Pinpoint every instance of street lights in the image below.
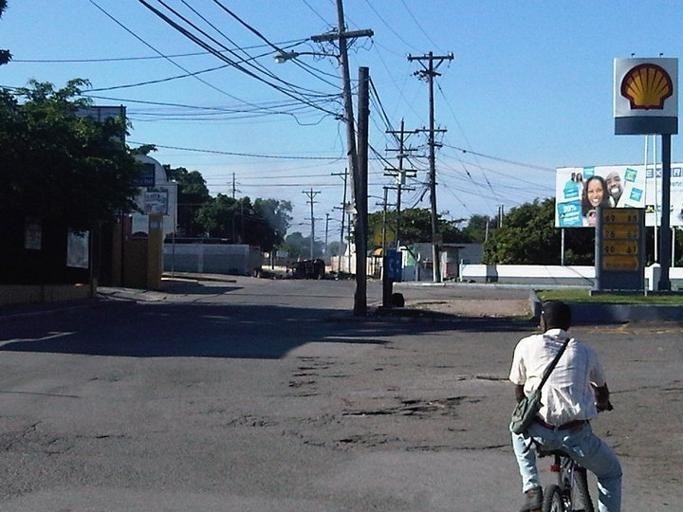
[274,51,368,316]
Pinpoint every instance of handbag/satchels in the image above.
[510,390,543,434]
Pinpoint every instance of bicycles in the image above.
[541,400,613,512]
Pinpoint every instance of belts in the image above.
[534,417,584,432]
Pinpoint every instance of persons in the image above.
[508,299,622,512]
[569,171,625,225]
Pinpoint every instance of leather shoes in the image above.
[519,486,543,512]
